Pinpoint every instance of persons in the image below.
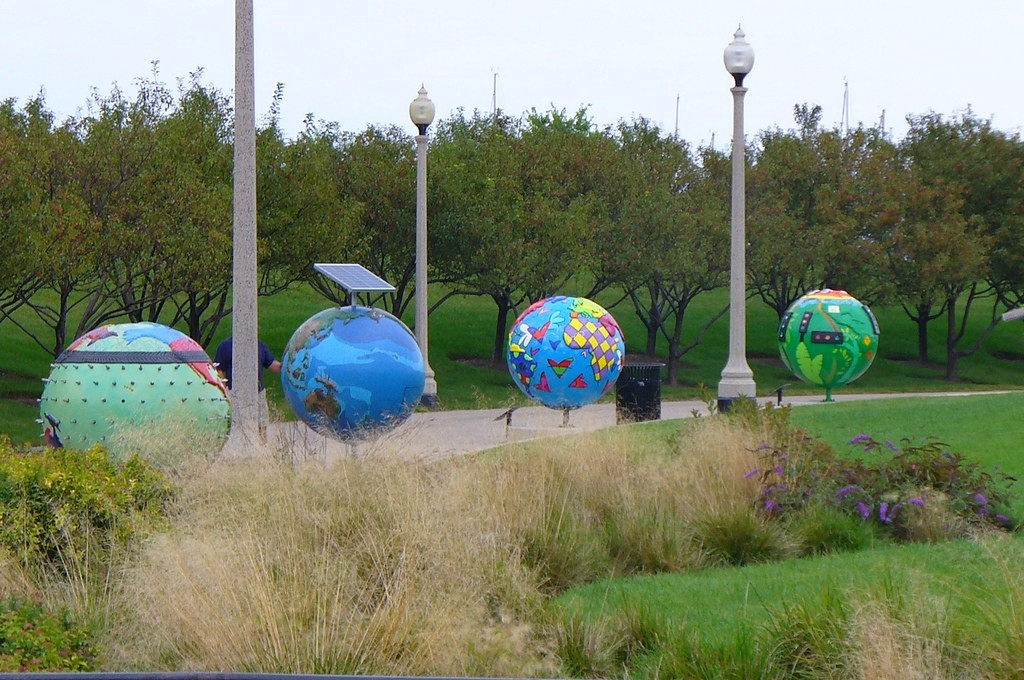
[214,334,281,444]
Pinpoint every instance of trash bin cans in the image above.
[613,362,666,423]
